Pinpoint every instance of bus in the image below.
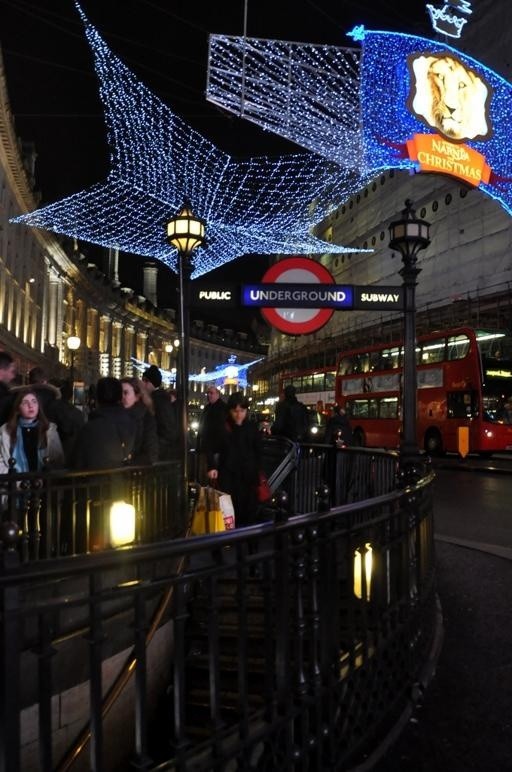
[280,365,336,417]
[336,328,512,460]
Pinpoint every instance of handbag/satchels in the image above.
[190,476,237,540]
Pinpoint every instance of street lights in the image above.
[387,196,430,486]
[166,195,204,536]
[67,328,81,397]
